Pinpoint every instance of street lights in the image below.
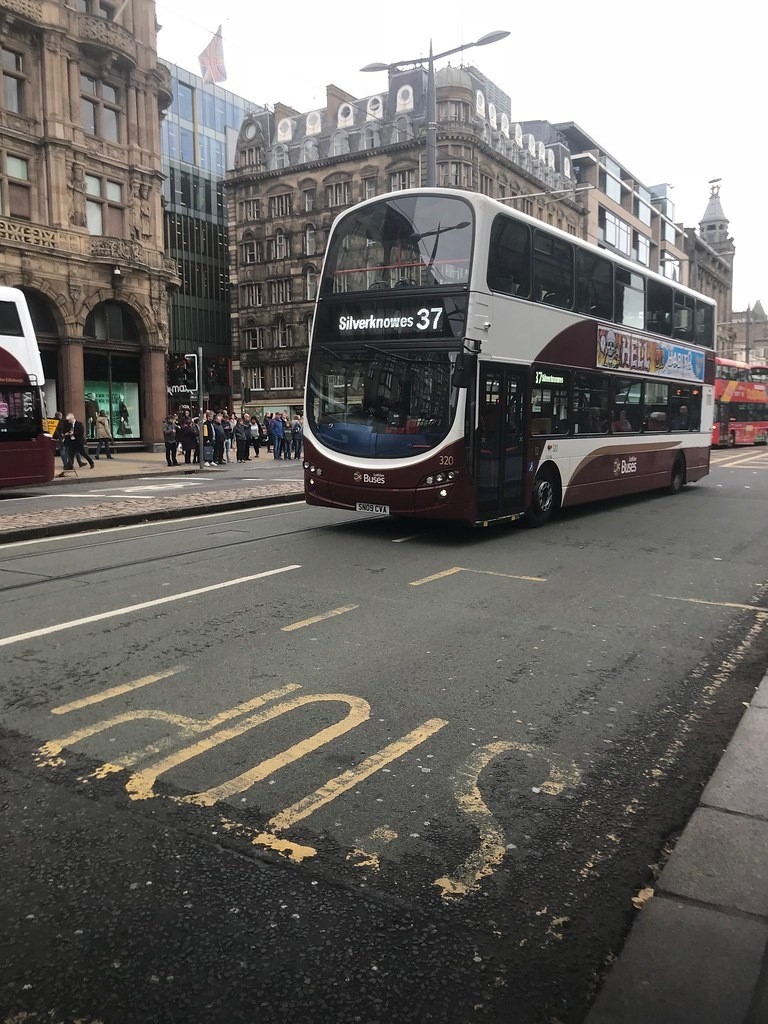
[658,251,734,292]
[409,221,471,284]
[358,29,511,186]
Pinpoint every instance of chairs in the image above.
[367,278,417,291]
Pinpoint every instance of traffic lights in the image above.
[183,353,198,391]
[204,369,215,391]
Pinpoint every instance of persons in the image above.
[88,393,100,438]
[52,411,94,470]
[117,395,128,435]
[95,409,115,460]
[640,405,660,431]
[582,407,632,433]
[647,308,670,334]
[673,406,696,430]
[162,409,304,467]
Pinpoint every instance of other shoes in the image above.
[204,462,210,466]
[90,462,94,469]
[242,460,245,463]
[238,460,241,463]
[255,456,259,457]
[227,460,234,463]
[79,463,87,467]
[64,467,73,469]
[95,456,99,459]
[248,459,251,460]
[210,462,217,466]
[219,462,226,464]
[107,456,113,459]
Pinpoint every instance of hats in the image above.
[276,412,282,415]
[54,412,61,418]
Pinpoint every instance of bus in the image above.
[533,396,580,412]
[670,356,768,448]
[0,286,55,490]
[302,185,718,528]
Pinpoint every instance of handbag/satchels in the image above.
[204,443,213,460]
